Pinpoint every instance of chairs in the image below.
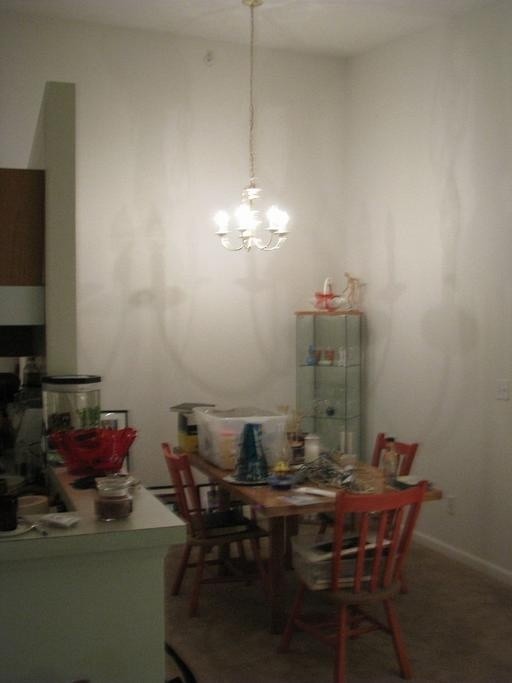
[282,480,433,682]
[159,445,272,617]
[314,432,420,597]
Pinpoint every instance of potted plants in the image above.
[98,409,131,477]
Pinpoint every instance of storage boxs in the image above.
[291,527,393,592]
[194,406,289,471]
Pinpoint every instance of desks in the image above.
[0,457,185,683]
[175,445,444,634]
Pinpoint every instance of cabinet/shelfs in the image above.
[90,474,137,522]
[294,309,362,459]
[377,437,398,484]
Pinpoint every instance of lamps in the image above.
[214,0,292,254]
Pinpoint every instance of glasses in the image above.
[214,0,292,254]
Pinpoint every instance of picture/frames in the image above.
[175,445,444,634]
[0,457,185,683]
[98,409,131,477]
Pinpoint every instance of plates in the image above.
[294,309,362,459]
